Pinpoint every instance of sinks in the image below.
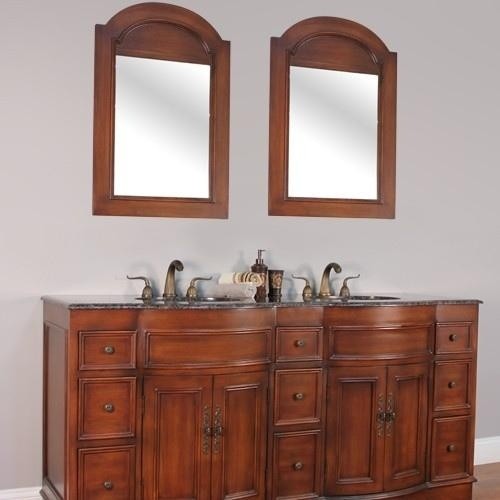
[135,297,241,302]
[335,296,401,300]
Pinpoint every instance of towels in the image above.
[209,282,257,298]
[219,272,263,288]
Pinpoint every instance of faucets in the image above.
[319,262,341,296]
[161,259,184,297]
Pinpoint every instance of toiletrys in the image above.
[251,249,268,303]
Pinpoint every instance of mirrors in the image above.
[91,2,232,220]
[267,14,398,220]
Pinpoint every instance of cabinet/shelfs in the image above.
[40,296,276,500]
[269,301,481,500]
